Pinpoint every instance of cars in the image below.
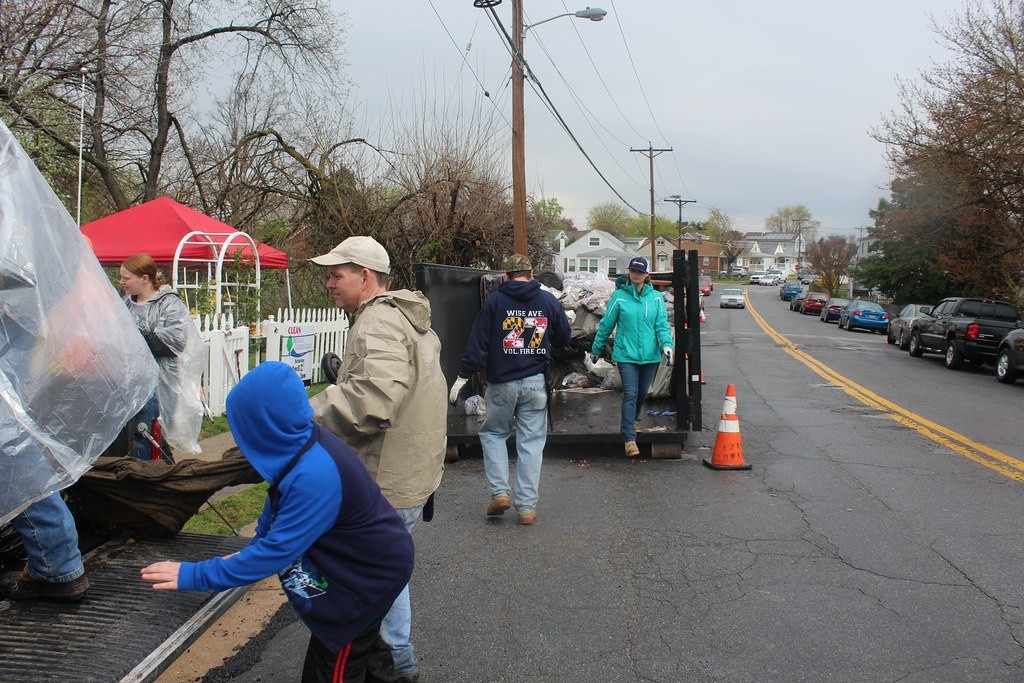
[887,303,937,351]
[750,268,788,286]
[801,274,820,285]
[799,292,830,315]
[719,289,747,309]
[995,320,1024,385]
[780,282,804,301]
[838,299,891,335]
[820,297,851,323]
[789,292,808,312]
[673,276,715,312]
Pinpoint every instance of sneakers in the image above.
[625,441,640,457]
[0,562,90,602]
[633,420,642,433]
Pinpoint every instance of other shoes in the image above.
[487,497,510,515]
[396,669,419,682]
[520,510,536,525]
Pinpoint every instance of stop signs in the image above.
[795,265,800,270]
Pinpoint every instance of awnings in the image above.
[79,196,295,332]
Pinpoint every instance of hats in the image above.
[505,254,532,272]
[626,257,648,273]
[309,236,391,275]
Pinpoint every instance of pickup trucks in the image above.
[909,296,1020,370]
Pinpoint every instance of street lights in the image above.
[512,0,608,261]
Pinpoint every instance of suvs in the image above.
[720,267,747,278]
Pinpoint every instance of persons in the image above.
[140,359,414,683]
[590,255,674,456]
[0,120,91,596]
[304,235,449,683]
[450,252,572,525]
[119,253,189,436]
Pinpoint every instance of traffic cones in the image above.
[703,383,753,470]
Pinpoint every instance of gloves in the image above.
[663,346,673,366]
[590,353,599,364]
[449,376,468,403]
